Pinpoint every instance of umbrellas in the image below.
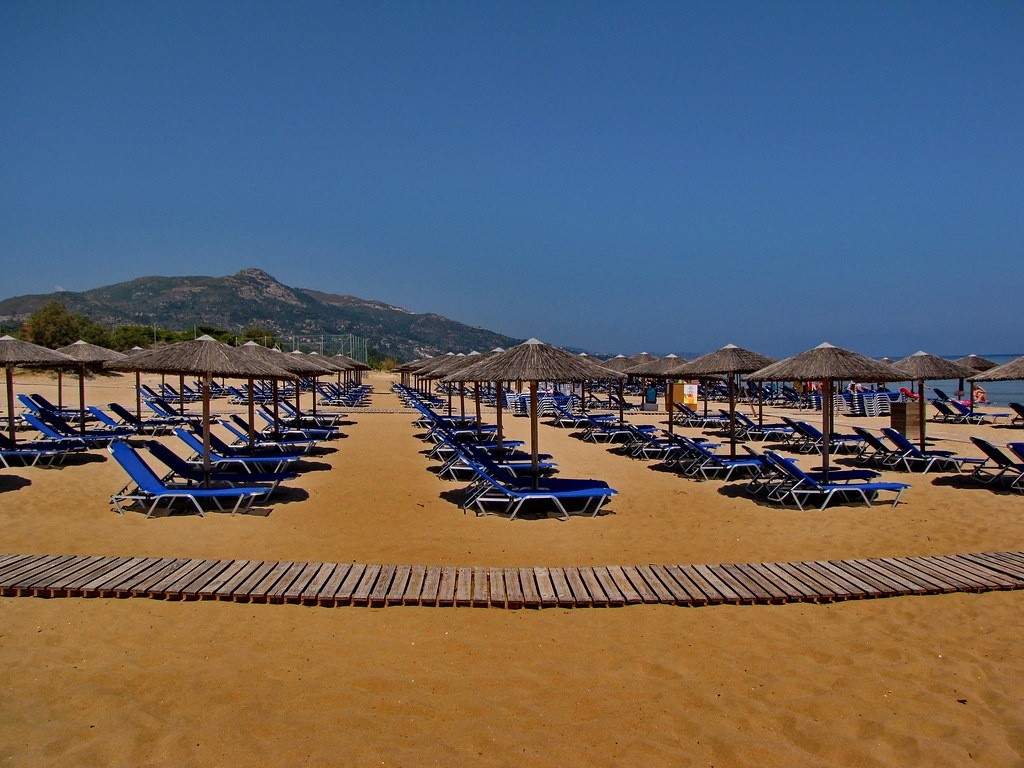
[967,357,1024,382]
[953,354,999,416]
[440,338,628,492]
[0,333,84,452]
[740,341,918,484]
[0,335,981,460]
[103,335,300,490]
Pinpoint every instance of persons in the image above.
[973,384,987,402]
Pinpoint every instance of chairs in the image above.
[0,378,374,520]
[390,381,1024,521]
